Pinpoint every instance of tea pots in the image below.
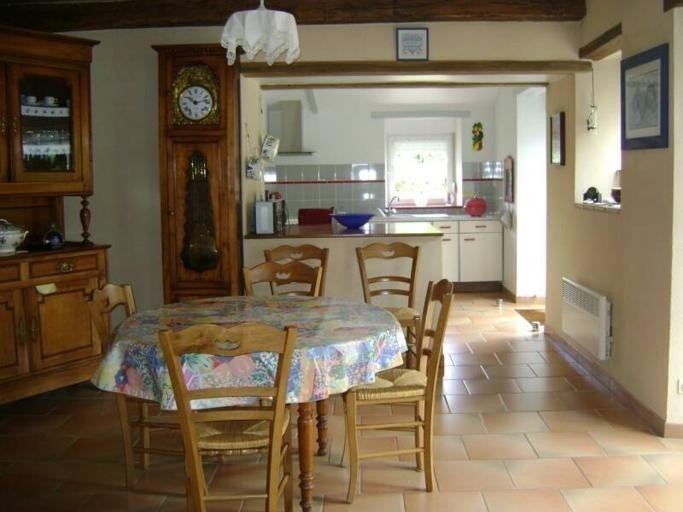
[0,220,28,255]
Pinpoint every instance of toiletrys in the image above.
[42,224,66,251]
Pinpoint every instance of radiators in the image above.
[559,273,611,364]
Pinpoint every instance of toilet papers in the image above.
[255,201,274,233]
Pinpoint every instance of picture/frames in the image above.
[394,26,429,62]
[548,110,566,168]
[616,42,672,151]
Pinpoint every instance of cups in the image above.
[20,93,72,108]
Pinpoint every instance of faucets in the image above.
[388,196,400,209]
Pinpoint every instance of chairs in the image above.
[355,243,422,369]
[262,245,329,297]
[157,321,297,512]
[242,261,323,297]
[82,283,188,489]
[338,278,455,503]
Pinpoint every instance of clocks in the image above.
[149,41,244,305]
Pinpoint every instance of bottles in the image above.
[22,126,71,172]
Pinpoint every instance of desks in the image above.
[90,298,408,512]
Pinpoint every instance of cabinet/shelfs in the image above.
[0,246,114,409]
[431,221,505,284]
[0,22,94,201]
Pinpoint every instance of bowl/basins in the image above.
[328,213,376,230]
[246,133,280,182]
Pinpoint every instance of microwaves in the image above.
[254,199,287,235]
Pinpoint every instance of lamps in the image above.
[219,1,300,66]
